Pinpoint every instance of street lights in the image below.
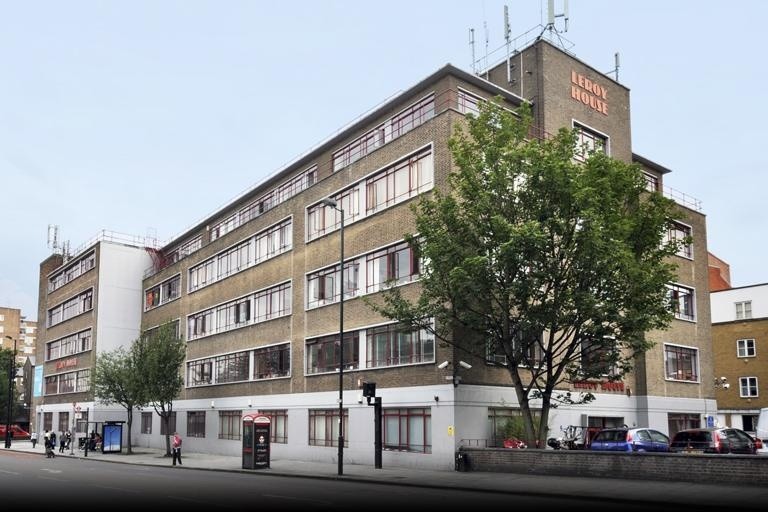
[4,335,18,449]
[69,400,76,456]
[319,194,350,475]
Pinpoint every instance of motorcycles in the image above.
[547,433,583,451]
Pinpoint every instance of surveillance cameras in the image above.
[721,377,727,381]
[723,384,730,388]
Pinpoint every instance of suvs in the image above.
[591,426,670,454]
[668,426,757,457]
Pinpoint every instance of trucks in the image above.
[755,406,768,457]
[1,424,32,442]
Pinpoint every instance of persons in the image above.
[30,428,102,458]
[170,432,183,467]
